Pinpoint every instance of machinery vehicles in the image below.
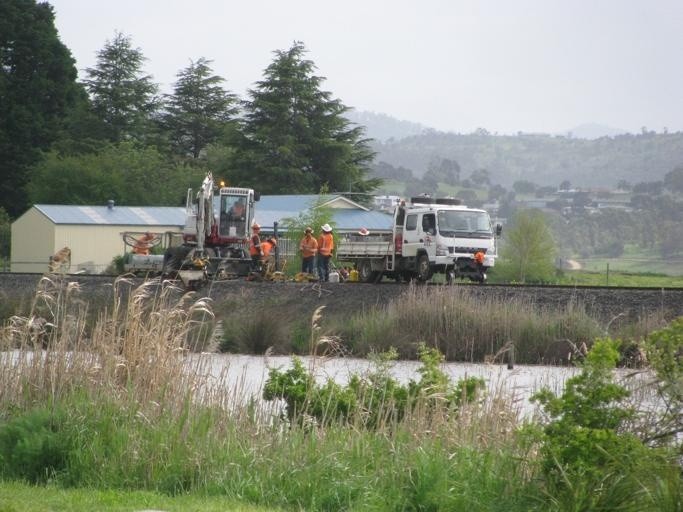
[162,169,260,290]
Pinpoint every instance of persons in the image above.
[227,197,245,222]
[316,224,335,283]
[329,266,359,283]
[130,231,155,255]
[298,227,317,274]
[249,223,277,282]
[474,249,485,283]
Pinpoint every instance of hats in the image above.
[321,224,332,233]
[304,227,315,234]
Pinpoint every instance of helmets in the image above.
[252,224,261,229]
[271,238,276,245]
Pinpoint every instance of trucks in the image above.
[334,201,502,285]
